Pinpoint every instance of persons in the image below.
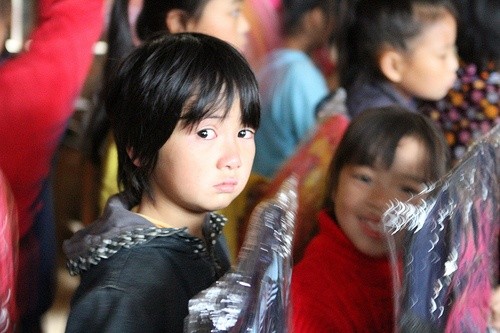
[417,0,500,179]
[66,29,262,333]
[81,0,253,229]
[248,0,340,178]
[0,0,108,333]
[340,0,459,123]
[286,105,499,332]
